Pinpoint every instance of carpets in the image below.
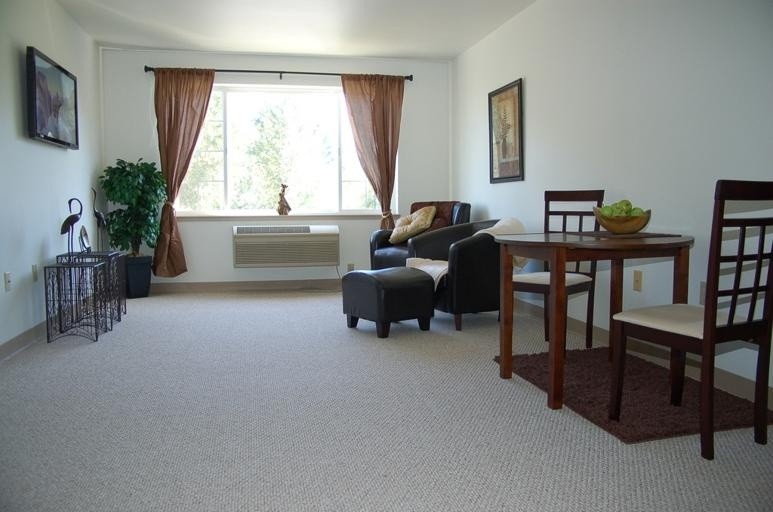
[494,346,773,445]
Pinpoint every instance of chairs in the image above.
[408,219,509,331]
[512,190,606,348]
[370,201,470,272]
[609,180,773,459]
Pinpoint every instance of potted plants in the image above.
[97,156,168,299]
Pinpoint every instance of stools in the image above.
[342,266,435,338]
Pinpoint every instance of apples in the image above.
[600,200,644,217]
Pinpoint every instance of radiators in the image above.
[233,225,340,268]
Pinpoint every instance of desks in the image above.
[494,231,693,410]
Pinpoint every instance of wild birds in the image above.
[61,198,82,256]
[91,187,106,253]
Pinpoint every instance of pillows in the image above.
[422,217,448,233]
[388,206,436,245]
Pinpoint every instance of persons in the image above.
[34,71,63,140]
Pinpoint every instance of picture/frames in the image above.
[488,77,525,181]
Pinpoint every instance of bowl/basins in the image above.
[593,206,651,234]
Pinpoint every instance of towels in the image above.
[405,217,531,293]
